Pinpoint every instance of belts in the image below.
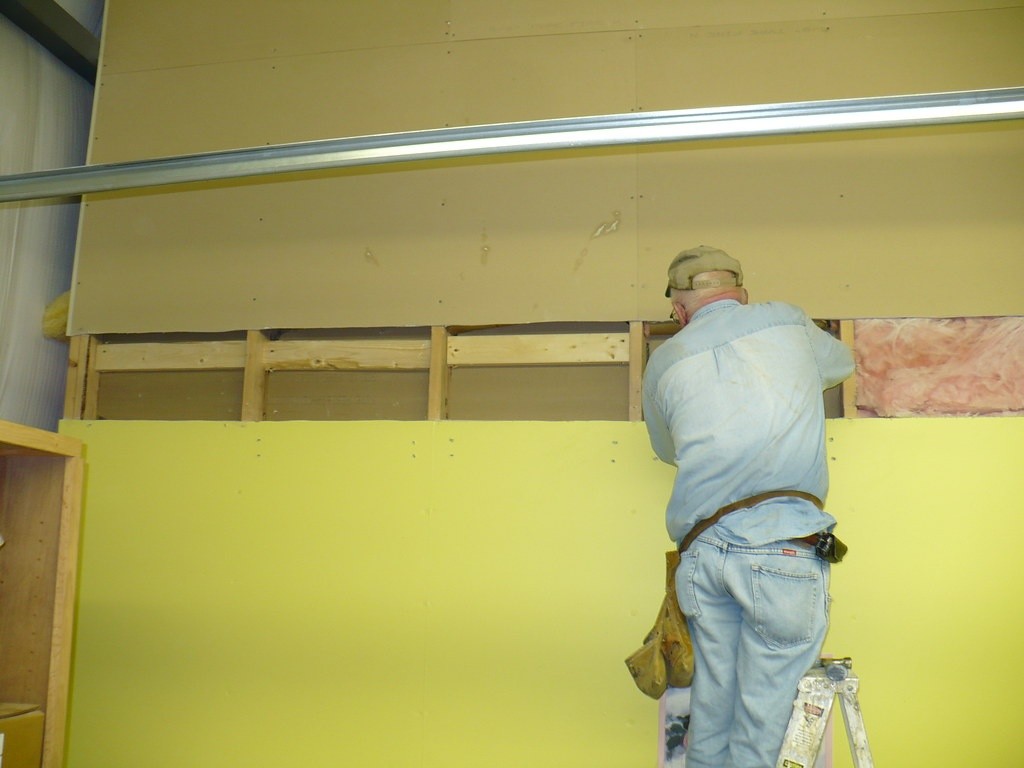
[795,533,819,545]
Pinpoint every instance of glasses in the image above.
[669,303,686,325]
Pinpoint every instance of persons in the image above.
[641,244,855,768]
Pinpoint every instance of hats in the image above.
[665,245,744,296]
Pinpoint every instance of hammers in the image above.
[811,657,853,681]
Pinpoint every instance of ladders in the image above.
[776,653,875,768]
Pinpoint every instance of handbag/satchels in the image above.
[625,550,694,699]
